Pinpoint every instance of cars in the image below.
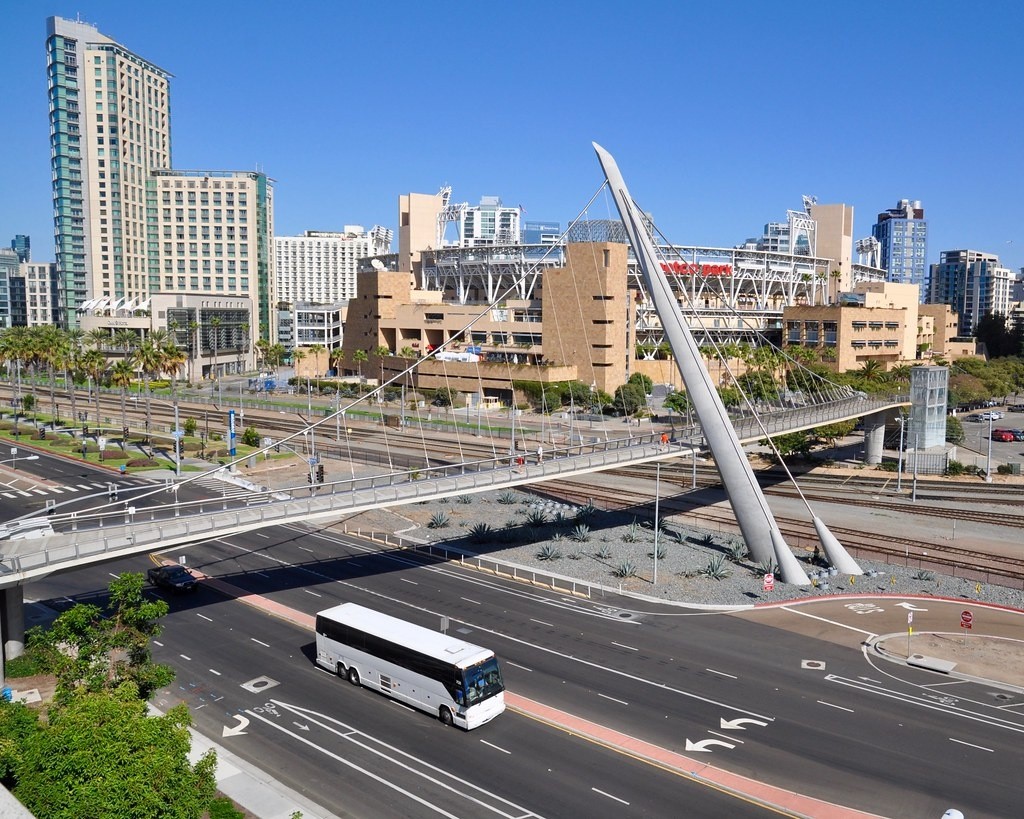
[948,399,1005,423]
[147,564,198,596]
[1009,429,1024,441]
[992,428,1013,441]
[1008,404,1024,413]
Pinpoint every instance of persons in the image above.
[535,444,546,467]
[659,432,670,452]
[517,454,525,474]
[477,675,488,690]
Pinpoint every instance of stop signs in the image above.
[961,611,974,624]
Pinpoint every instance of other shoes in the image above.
[537,464,539,466]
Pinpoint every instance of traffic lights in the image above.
[180,438,184,453]
[307,471,311,484]
[318,464,324,484]
[124,427,129,438]
[173,442,176,453]
[40,428,46,440]
[316,472,319,481]
[84,425,88,437]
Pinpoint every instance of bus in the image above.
[315,602,507,733]
